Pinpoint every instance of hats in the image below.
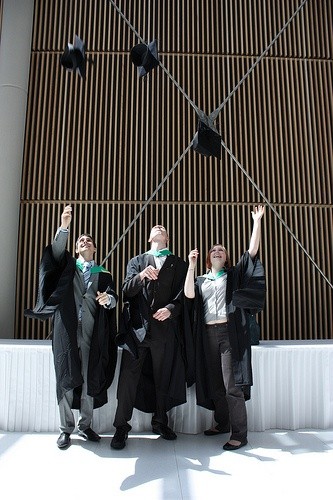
[131,40,159,78]
[193,122,222,159]
[59,34,86,78]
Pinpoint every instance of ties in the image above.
[82,262,91,285]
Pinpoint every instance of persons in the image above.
[24,204,119,448]
[184,205,265,450]
[110,224,195,450]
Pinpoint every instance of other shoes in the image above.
[78,428,101,441]
[111,428,128,449]
[204,426,230,435]
[56,432,70,448]
[223,438,248,450]
[152,424,178,440]
